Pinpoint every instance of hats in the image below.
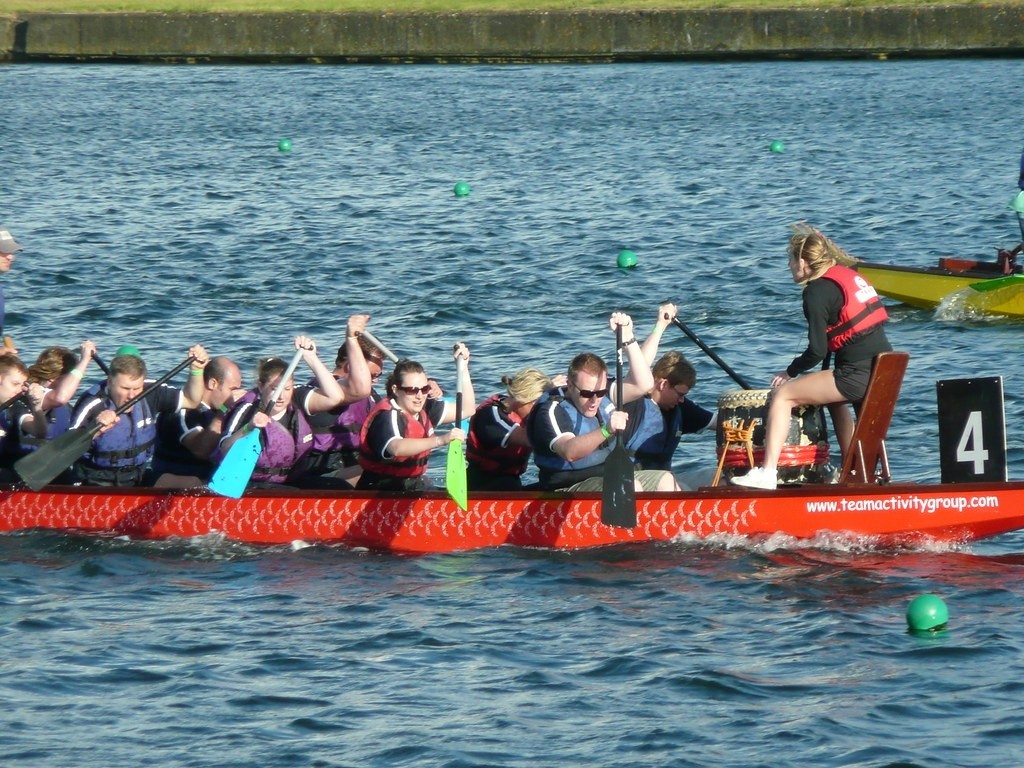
[0,228,23,254]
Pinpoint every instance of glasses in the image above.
[397,385,430,395]
[572,382,607,398]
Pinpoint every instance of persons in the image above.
[465,303,720,495]
[1004,146,1024,274]
[730,221,892,490]
[0,315,476,492]
[0,224,23,356]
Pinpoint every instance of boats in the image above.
[850,258,1024,318]
[0,482,1024,557]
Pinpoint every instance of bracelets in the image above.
[220,404,227,413]
[621,337,635,347]
[70,368,83,379]
[190,366,204,375]
[602,422,613,440]
[244,423,251,433]
[346,336,358,339]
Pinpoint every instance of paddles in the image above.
[13,353,205,492]
[664,313,752,390]
[355,317,480,435]
[444,345,467,511]
[91,350,110,375]
[0,381,31,413]
[208,345,314,499]
[600,322,637,528]
[969,274,1024,293]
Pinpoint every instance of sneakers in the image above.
[730,467,778,490]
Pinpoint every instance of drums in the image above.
[716,389,830,485]
